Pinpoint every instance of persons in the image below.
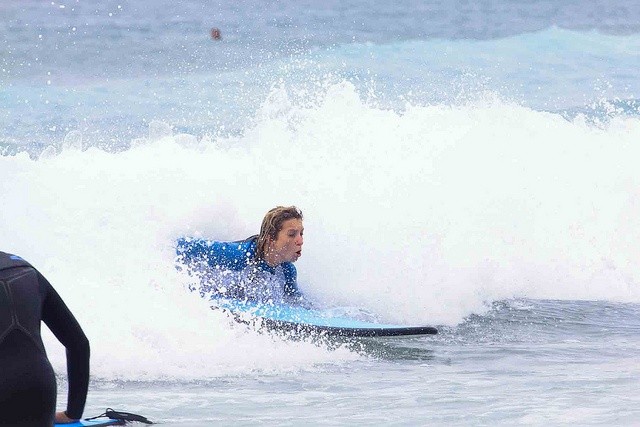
[173,204,312,320]
[0,250,90,427]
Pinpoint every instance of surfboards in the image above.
[52,416,127,427]
[220,301,438,336]
